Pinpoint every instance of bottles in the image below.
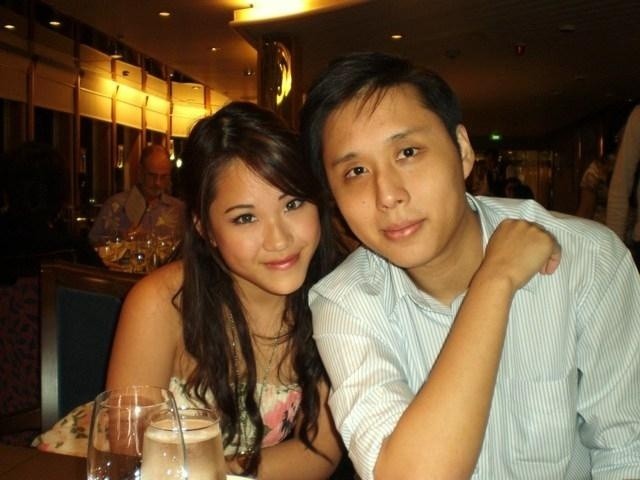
[102,230,174,273]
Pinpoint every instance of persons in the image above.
[573,124,636,238]
[296,48,639,479]
[30,99,349,479]
[88,143,187,250]
[498,163,535,202]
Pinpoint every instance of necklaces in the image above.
[229,312,287,469]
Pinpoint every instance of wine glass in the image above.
[140,407,226,480]
[86,384,188,480]
